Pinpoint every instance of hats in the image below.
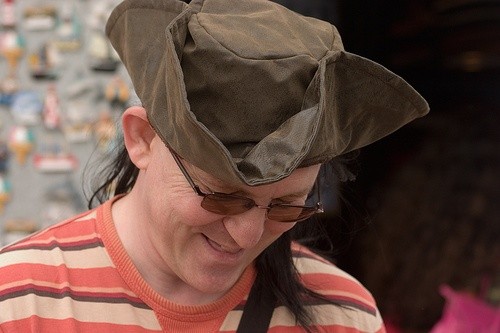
[103,1,431,189]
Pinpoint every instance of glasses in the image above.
[164,145,324,222]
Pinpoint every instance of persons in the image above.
[370,253,499,332]
[0,1,394,333]
